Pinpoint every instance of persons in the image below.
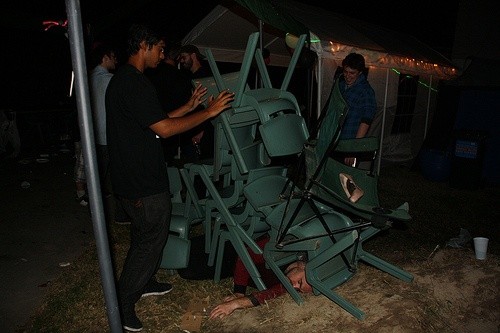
[68,82,89,207]
[90,50,134,227]
[261,49,270,65]
[178,231,312,320]
[105,24,235,330]
[163,40,179,75]
[180,45,213,201]
[329,52,376,168]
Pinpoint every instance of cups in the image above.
[474,237,489,260]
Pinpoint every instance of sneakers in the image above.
[102,192,112,199]
[140,282,173,297]
[119,310,143,331]
[114,216,132,224]
[76,194,91,208]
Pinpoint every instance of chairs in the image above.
[160,32,413,320]
